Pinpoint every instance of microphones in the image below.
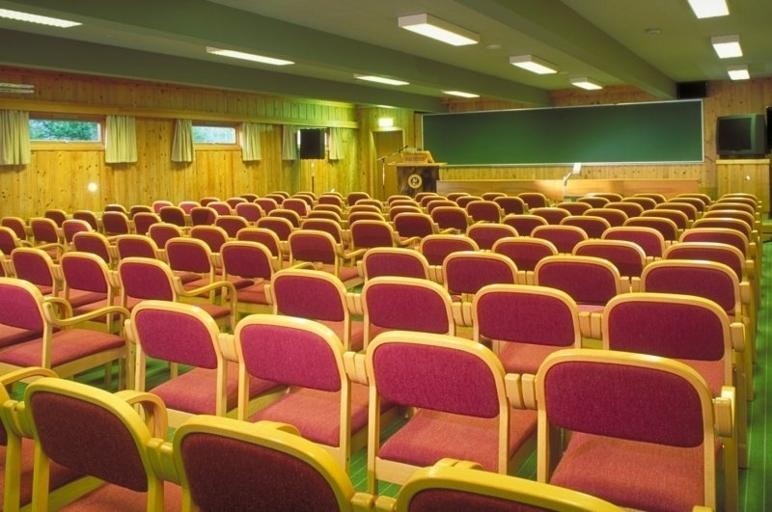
[397,144,409,154]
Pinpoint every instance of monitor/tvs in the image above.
[716,113,768,159]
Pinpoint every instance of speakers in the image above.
[300,128,325,159]
[677,81,710,99]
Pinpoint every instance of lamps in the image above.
[397,13,481,48]
[711,35,744,59]
[508,54,560,76]
[728,65,750,81]
[569,77,605,91]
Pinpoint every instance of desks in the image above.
[435,177,702,208]
[387,162,448,197]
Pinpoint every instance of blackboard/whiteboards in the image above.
[422,98,705,168]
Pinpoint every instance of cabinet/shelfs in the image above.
[713,159,771,219]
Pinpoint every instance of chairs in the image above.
[254,216,295,241]
[621,197,657,211]
[104,204,127,214]
[685,217,762,299]
[528,206,571,226]
[200,197,219,206]
[426,199,458,214]
[590,292,749,473]
[307,209,341,223]
[1,225,31,275]
[220,239,316,313]
[708,202,763,264]
[0,363,62,511]
[669,197,705,214]
[286,230,366,292]
[414,234,484,266]
[493,197,529,217]
[282,198,309,215]
[263,268,379,351]
[100,211,129,235]
[71,209,97,230]
[236,227,291,272]
[374,458,627,512]
[355,275,463,350]
[441,252,526,302]
[463,284,589,379]
[178,202,200,215]
[2,215,28,245]
[531,225,587,255]
[9,248,63,296]
[152,201,172,212]
[578,198,609,210]
[572,238,654,278]
[239,195,257,203]
[501,214,548,238]
[390,212,461,248]
[162,238,254,299]
[631,193,665,205]
[389,206,422,221]
[603,202,645,219]
[163,415,374,512]
[266,209,301,229]
[432,206,487,236]
[517,192,551,212]
[146,222,184,251]
[56,251,144,332]
[129,205,152,217]
[113,256,239,338]
[207,202,232,215]
[124,299,286,430]
[467,224,519,251]
[630,258,753,399]
[356,330,537,497]
[188,206,219,227]
[225,198,249,209]
[317,197,342,207]
[0,278,129,393]
[214,215,251,237]
[559,215,611,238]
[662,241,759,348]
[300,217,346,256]
[654,201,702,222]
[467,201,508,225]
[313,204,345,218]
[253,197,278,216]
[159,206,186,226]
[527,255,630,314]
[345,192,369,207]
[622,216,684,242]
[672,227,759,320]
[111,235,203,286]
[639,208,694,231]
[44,209,67,228]
[601,225,670,265]
[346,219,422,266]
[187,224,230,253]
[348,205,382,212]
[591,193,623,204]
[348,211,387,229]
[292,195,314,210]
[61,220,93,248]
[676,193,712,206]
[67,230,115,269]
[486,237,565,272]
[701,210,762,278]
[389,205,423,222]
[356,246,462,303]
[265,194,285,204]
[557,201,592,215]
[227,312,398,475]
[132,211,160,234]
[25,218,64,259]
[16,375,168,511]
[633,194,646,200]
[235,204,264,225]
[582,208,628,228]
[521,347,740,511]
